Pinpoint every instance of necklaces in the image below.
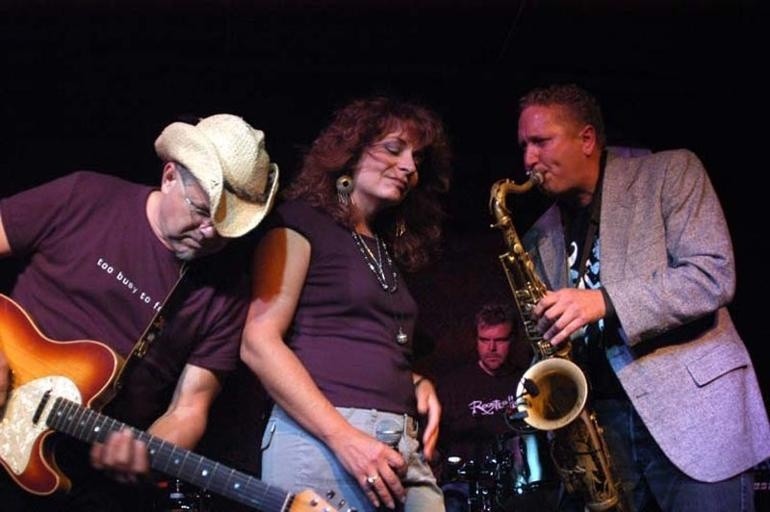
[350,223,409,345]
[350,230,400,295]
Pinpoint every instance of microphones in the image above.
[503,379,539,434]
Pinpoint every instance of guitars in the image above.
[0,293,357,512]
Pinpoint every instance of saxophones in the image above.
[489,170,634,512]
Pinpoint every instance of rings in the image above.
[366,475,379,483]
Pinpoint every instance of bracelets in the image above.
[414,376,425,388]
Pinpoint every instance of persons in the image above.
[0,114,281,512]
[240,96,453,511]
[431,302,525,511]
[517,82,770,511]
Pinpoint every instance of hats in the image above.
[150,112,285,245]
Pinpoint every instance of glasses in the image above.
[169,166,210,221]
